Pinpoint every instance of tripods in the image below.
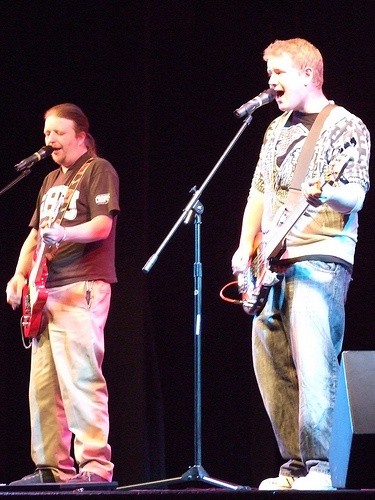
[116,115,252,490]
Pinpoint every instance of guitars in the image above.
[238,144,356,315]
[21,191,61,340]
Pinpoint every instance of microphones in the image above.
[14,145,54,171]
[234,89,276,119]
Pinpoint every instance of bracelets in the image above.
[56,228,65,244]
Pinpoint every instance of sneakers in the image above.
[68,471,109,483]
[258,474,299,490]
[292,471,337,491]
[9,469,56,485]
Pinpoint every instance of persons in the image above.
[231,38,370,491]
[5,103,121,486]
